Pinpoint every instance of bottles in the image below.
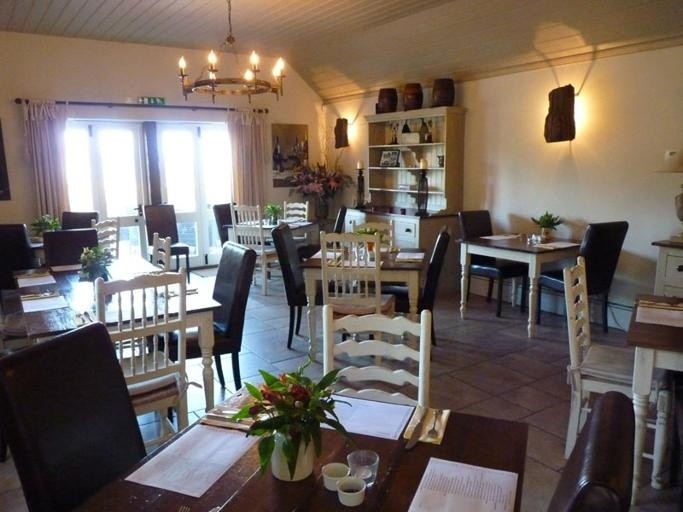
[400,119,432,144]
[263,219,270,225]
[419,159,427,169]
[526,232,541,245]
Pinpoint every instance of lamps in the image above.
[178,0,286,104]
[650,148,683,224]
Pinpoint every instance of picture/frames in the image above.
[378,149,400,168]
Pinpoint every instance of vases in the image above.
[270,429,315,482]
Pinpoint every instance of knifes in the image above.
[405,408,431,449]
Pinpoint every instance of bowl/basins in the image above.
[665,151,683,171]
[376,247,399,261]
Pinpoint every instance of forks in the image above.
[426,409,442,439]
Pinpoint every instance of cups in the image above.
[375,78,454,114]
[371,206,405,214]
[347,448,380,487]
[322,462,350,491]
[436,155,443,166]
[335,476,366,508]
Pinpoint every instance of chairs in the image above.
[0,323,147,512]
[547,391,636,512]
[564,255,672,491]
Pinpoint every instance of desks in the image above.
[626,293,683,507]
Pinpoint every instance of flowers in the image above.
[229,354,359,483]
[284,163,355,205]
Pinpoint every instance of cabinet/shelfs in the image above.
[651,237,683,298]
[343,106,467,295]
